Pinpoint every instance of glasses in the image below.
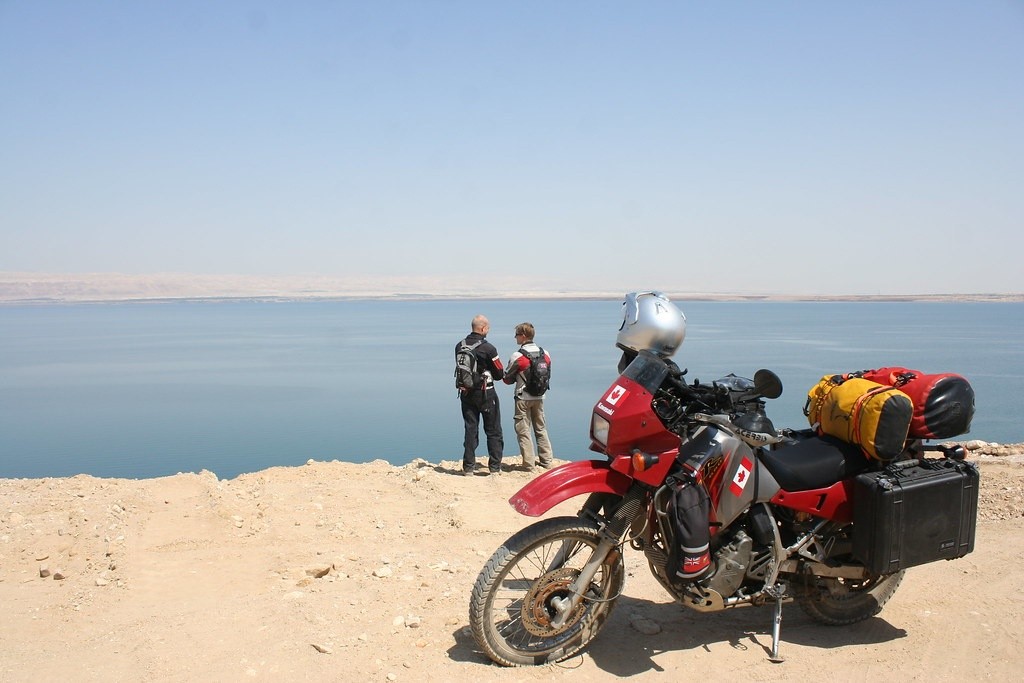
[515,333,522,336]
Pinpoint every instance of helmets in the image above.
[615,289,687,358]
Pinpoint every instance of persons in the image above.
[454,313,503,476]
[502,322,552,469]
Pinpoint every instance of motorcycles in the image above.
[469,351,980,667]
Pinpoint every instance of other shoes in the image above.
[490,469,503,477]
[463,470,473,476]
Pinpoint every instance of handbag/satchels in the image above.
[806,364,975,458]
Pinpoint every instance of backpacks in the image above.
[453,339,488,406]
[519,347,551,397]
